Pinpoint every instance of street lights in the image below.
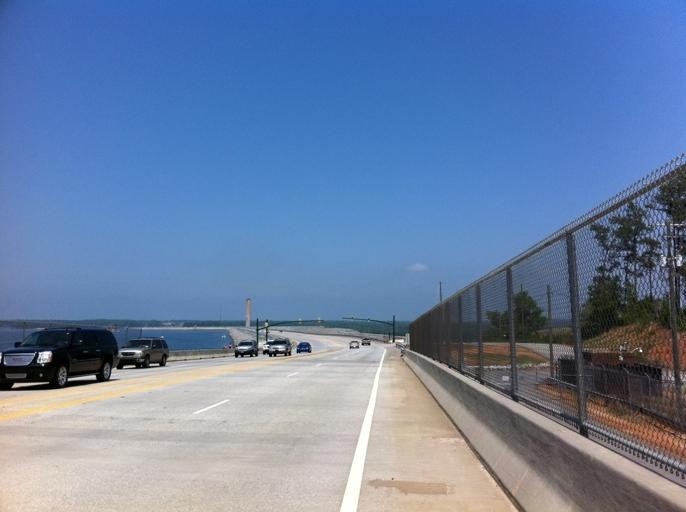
[657,220,686,432]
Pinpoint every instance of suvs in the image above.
[0,326,169,389]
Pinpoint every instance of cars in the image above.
[235,338,313,357]
[349,337,371,349]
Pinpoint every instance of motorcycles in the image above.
[398,344,407,357]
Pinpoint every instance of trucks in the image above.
[557,351,662,396]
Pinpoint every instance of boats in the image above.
[103,323,120,334]
